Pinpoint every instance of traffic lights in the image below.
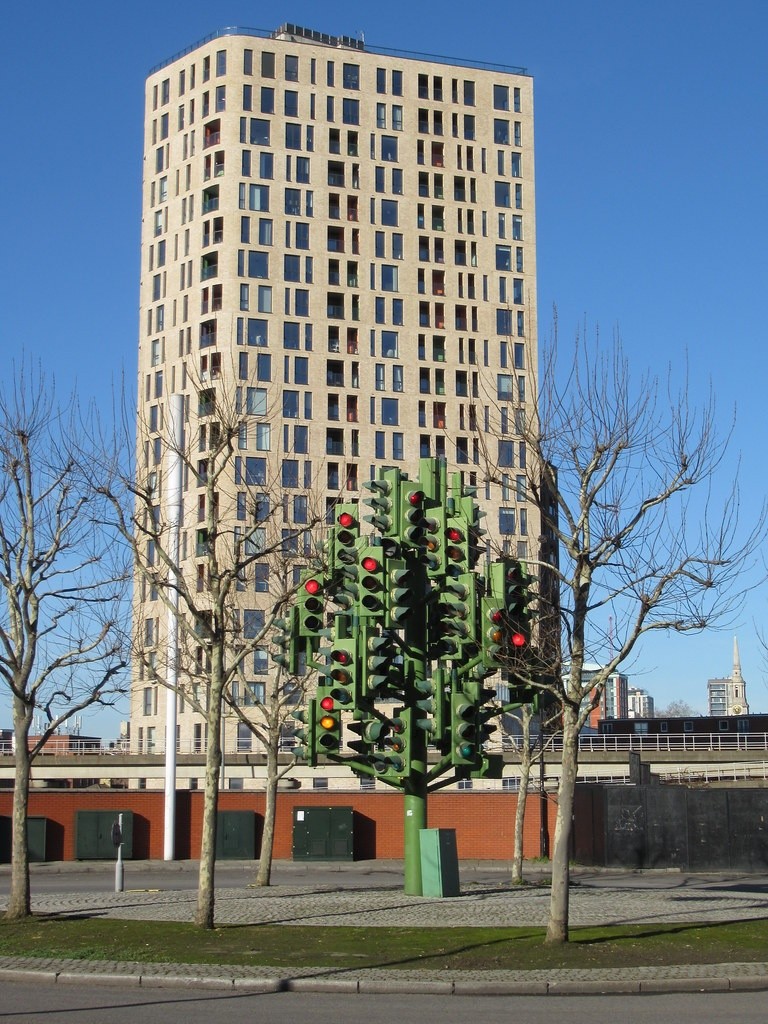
[449,692,478,769]
[271,606,297,676]
[313,687,346,758]
[459,680,497,745]
[288,699,316,767]
[300,568,325,639]
[310,451,542,755]
[416,666,448,747]
[368,706,413,782]
